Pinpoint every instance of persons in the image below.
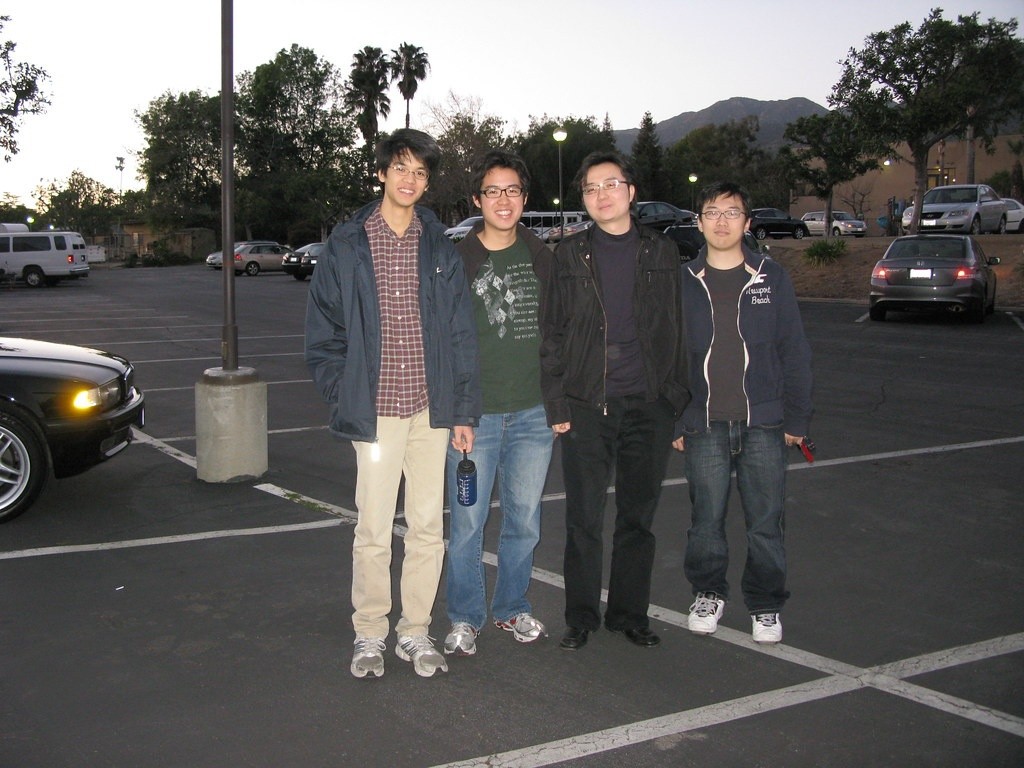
[444,152,559,655]
[672,181,812,644]
[305,128,482,677]
[540,152,691,649]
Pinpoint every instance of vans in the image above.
[0,223,92,289]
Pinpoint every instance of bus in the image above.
[519,210,595,244]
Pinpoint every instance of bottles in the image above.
[456,449,477,507]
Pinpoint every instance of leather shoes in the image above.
[559,623,594,650]
[604,617,660,648]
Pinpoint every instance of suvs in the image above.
[662,216,771,265]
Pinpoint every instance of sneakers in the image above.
[688,596,725,635]
[443,620,481,657]
[493,611,547,645]
[350,630,387,679]
[751,611,782,643]
[395,633,448,678]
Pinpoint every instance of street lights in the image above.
[688,172,699,212]
[552,197,560,227]
[552,125,569,243]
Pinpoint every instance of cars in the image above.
[0,336,147,525]
[281,242,325,282]
[206,241,281,272]
[800,210,869,238]
[443,215,483,244]
[868,233,1003,325]
[749,207,808,241]
[901,183,1009,236]
[233,243,296,277]
[630,201,699,233]
[980,197,1024,235]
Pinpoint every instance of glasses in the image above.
[583,178,630,194]
[700,209,748,221]
[476,187,526,198]
[386,164,430,180]
